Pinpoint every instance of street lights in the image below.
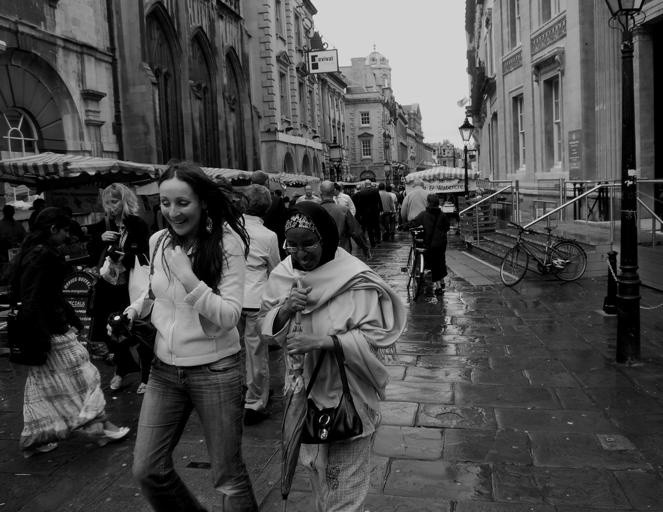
[605,0,651,368]
[380,158,394,192]
[458,113,476,200]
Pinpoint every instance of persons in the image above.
[0,196,84,254]
[399,177,430,223]
[86,181,150,394]
[226,186,281,425]
[255,201,408,511]
[398,194,450,295]
[252,172,406,258]
[2,206,131,460]
[106,158,257,510]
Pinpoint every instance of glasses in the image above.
[282,239,319,253]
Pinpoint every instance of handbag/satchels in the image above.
[128,253,151,305]
[7,312,47,365]
[99,244,129,285]
[299,335,363,443]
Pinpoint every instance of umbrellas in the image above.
[280,279,310,511]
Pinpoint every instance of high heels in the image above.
[97,426,130,446]
[24,442,58,458]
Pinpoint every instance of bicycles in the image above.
[496,214,587,286]
[404,230,437,301]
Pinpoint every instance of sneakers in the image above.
[110,375,122,390]
[137,383,147,394]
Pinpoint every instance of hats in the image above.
[36,206,72,228]
